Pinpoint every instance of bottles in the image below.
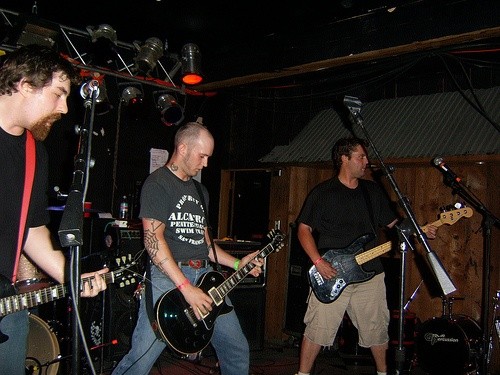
[119,195,128,220]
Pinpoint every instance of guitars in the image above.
[309,206,473,305]
[153,229,285,358]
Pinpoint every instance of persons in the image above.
[293,138,437,375]
[0,43,109,375]
[111,122,266,375]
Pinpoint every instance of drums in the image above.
[385,310,417,345]
[417,314,482,375]
[26,313,60,375]
[0,253,139,344]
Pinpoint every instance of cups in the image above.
[84,202,92,217]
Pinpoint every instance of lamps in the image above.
[132,35,168,72]
[152,89,185,127]
[180,42,203,85]
[86,22,119,55]
[120,86,144,107]
[80,79,107,212]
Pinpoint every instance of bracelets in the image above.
[233,259,241,271]
[314,257,324,266]
[177,280,189,289]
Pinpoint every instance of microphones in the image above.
[80,80,100,99]
[431,156,462,183]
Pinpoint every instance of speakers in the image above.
[101,226,144,361]
